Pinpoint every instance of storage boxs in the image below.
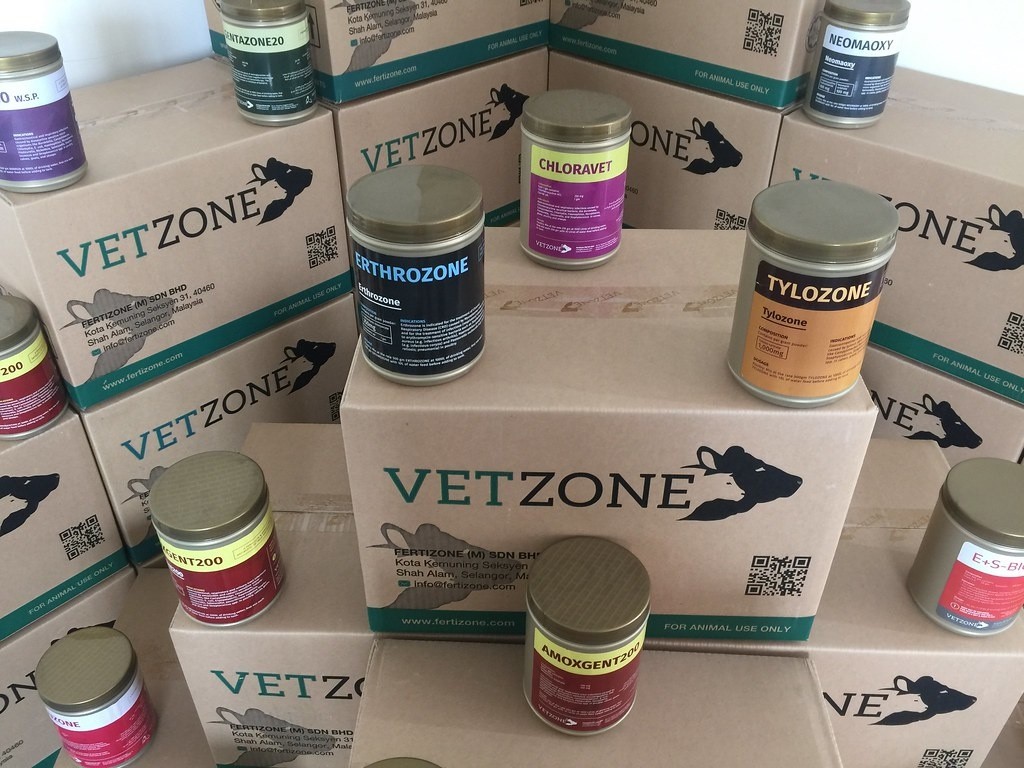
[0,0,1024,768]
